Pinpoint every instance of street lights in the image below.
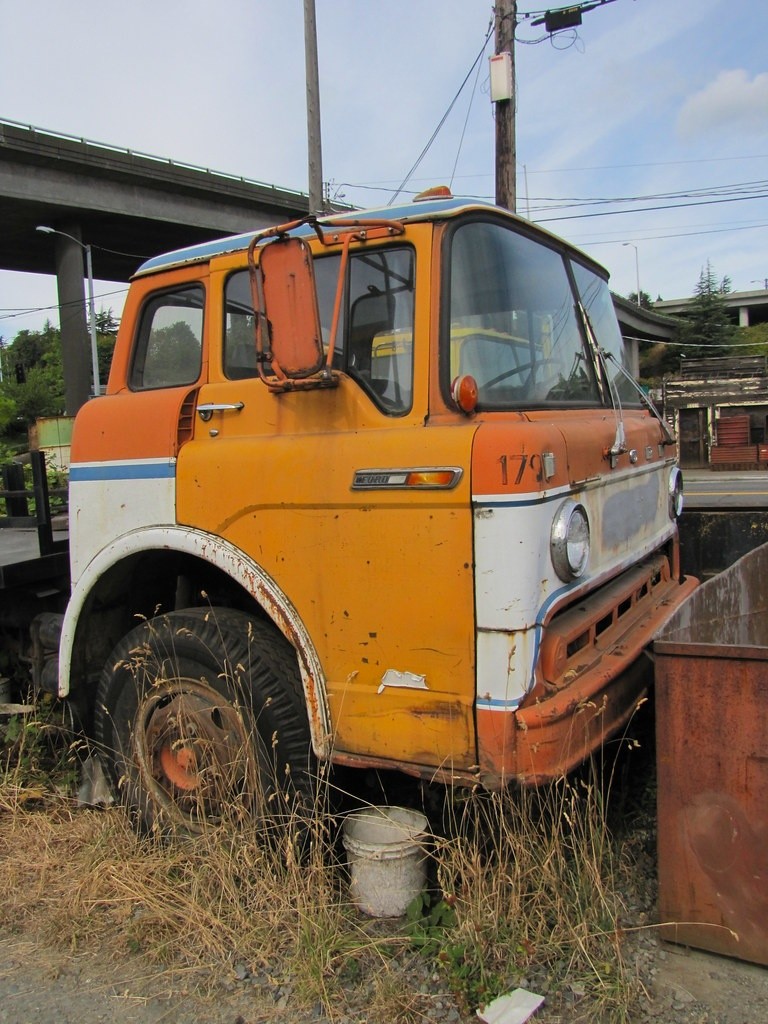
[621,242,641,309]
[35,225,102,399]
[750,280,767,291]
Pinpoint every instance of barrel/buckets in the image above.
[343,806,431,919]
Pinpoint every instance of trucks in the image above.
[0,188,699,875]
[310,329,550,398]
